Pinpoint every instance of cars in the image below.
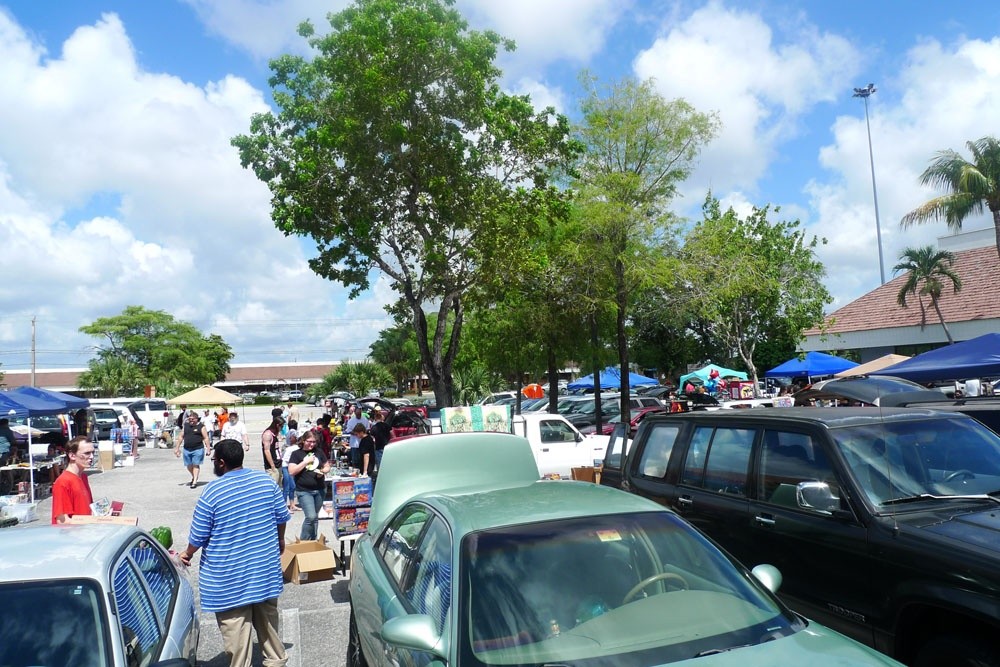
[347,432,906,667]
[539,379,571,396]
[325,391,427,446]
[0,524,202,667]
[304,394,324,408]
[229,389,303,404]
[476,388,672,436]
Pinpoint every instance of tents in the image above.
[679,364,748,394]
[567,367,660,395]
[765,332,1000,391]
[167,386,245,424]
[0,385,104,502]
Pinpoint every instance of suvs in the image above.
[820,373,1000,436]
[601,407,1000,667]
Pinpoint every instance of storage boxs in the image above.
[2,500,42,522]
[332,508,370,536]
[98,451,115,469]
[280,540,343,583]
[333,477,373,507]
[98,440,115,450]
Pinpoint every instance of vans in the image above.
[12,414,69,447]
[72,400,145,442]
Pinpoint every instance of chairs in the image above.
[852,435,900,503]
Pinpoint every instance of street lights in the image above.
[852,81,886,285]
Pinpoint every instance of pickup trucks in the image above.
[415,412,637,481]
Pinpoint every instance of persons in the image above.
[288,432,331,541]
[984,379,995,396]
[221,412,249,452]
[214,407,230,431]
[178,405,189,429]
[956,390,962,396]
[52,436,93,525]
[965,379,984,397]
[178,439,291,667]
[175,412,210,488]
[202,409,215,449]
[0,442,22,495]
[779,385,794,397]
[332,402,395,478]
[262,403,337,515]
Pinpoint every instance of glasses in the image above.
[212,456,227,464]
[306,438,317,445]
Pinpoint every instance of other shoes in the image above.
[190,477,198,486]
[288,510,294,514]
[290,506,302,511]
[190,484,197,488]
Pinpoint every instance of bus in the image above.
[84,397,173,438]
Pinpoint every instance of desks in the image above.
[317,501,363,576]
[144,436,160,448]
[0,454,66,495]
[325,473,359,500]
